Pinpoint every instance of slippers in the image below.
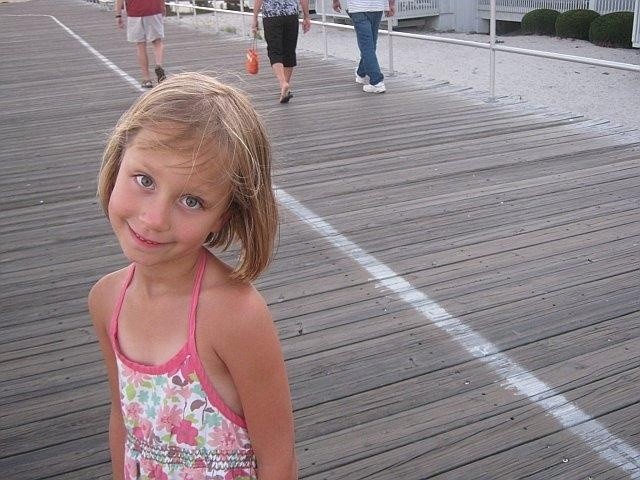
[280,90,293,102]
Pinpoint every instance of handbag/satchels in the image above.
[247,49,258,74]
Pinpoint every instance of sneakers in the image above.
[356,68,364,83]
[155,68,166,83]
[141,81,153,88]
[363,81,386,93]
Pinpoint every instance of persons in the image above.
[86,71,299,480]
[250,0,311,104]
[115,0,168,89]
[332,0,395,93]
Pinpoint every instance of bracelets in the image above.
[115,15,121,18]
